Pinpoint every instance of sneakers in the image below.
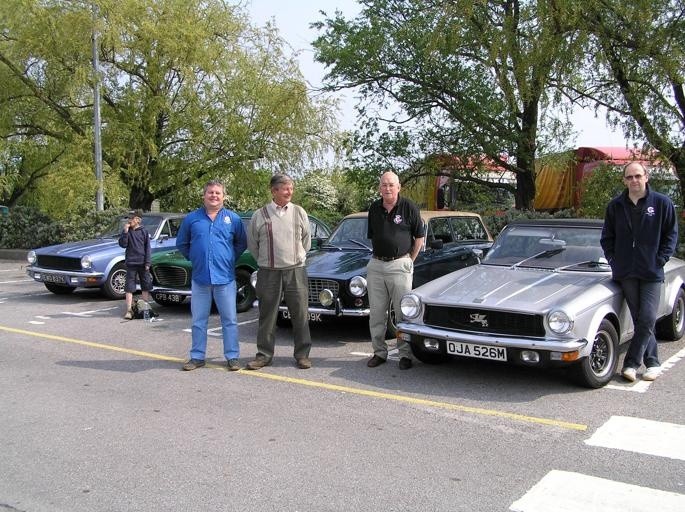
[621,367,636,382]
[124,311,133,319]
[247,353,272,369]
[228,358,240,370]
[183,358,204,370]
[642,366,661,380]
[399,357,409,370]
[297,358,311,369]
[368,355,386,367]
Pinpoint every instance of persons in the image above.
[600,162,678,381]
[176,179,246,371]
[437,183,449,209]
[119,210,151,320]
[247,174,312,369]
[366,172,424,369]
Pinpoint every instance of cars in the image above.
[24,211,186,300]
[396,218,685,389]
[151,209,337,315]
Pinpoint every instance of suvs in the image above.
[249,207,493,338]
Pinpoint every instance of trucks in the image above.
[532,147,684,219]
[397,152,516,216]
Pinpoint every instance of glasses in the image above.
[624,175,645,180]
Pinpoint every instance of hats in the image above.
[124,210,142,218]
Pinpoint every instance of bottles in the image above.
[143,302,150,322]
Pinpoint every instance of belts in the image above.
[373,255,401,261]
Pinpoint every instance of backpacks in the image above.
[133,299,152,319]
